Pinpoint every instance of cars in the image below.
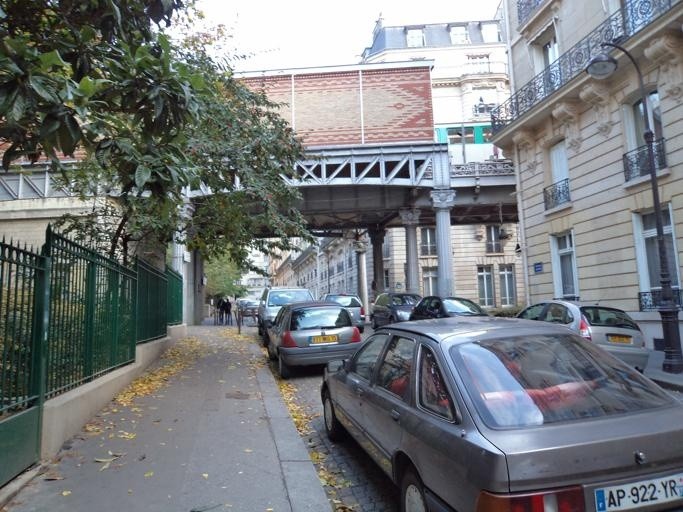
[321,312,683,512]
[265,300,362,380]
[408,296,488,320]
[368,290,423,329]
[513,298,651,373]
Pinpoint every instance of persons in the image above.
[208,294,231,325]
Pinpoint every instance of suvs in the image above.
[320,293,366,333]
[257,286,316,347]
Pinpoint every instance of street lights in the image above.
[585,40,682,375]
